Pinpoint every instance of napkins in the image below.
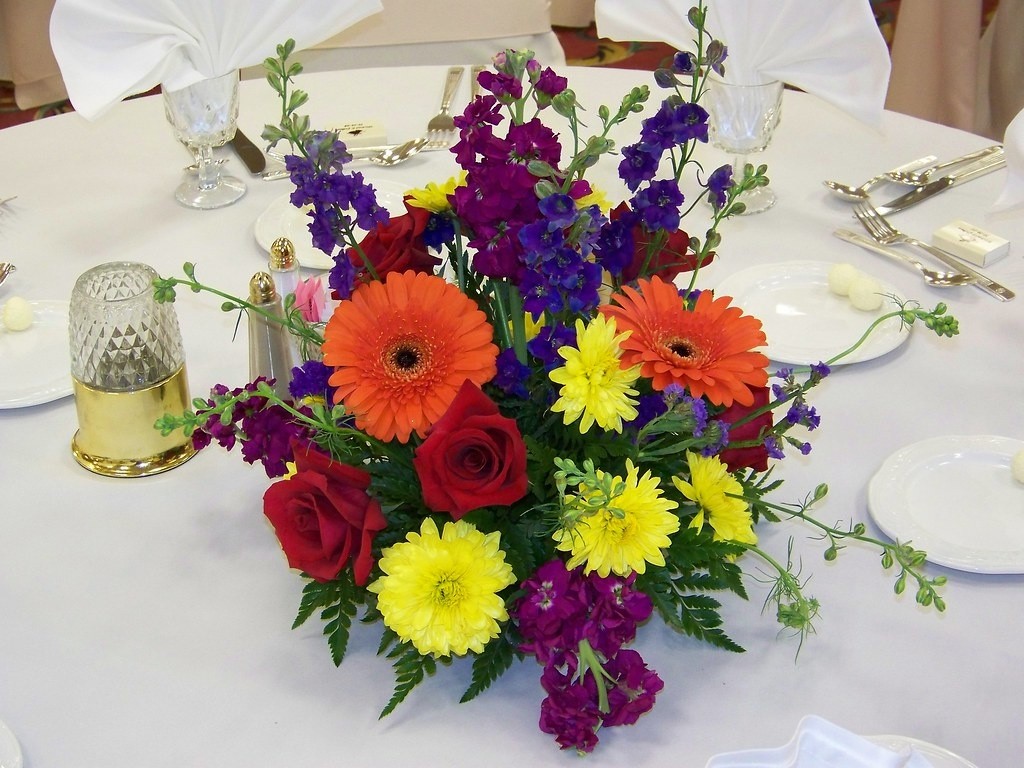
[49,0,387,121]
[593,1,893,128]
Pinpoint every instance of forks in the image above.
[263,140,452,166]
[851,197,1018,303]
[426,65,466,149]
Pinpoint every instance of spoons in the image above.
[182,145,231,176]
[832,227,978,289]
[883,145,1004,187]
[260,137,430,182]
[819,154,939,202]
[0,262,13,287]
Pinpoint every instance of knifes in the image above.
[231,129,266,175]
[852,151,1006,219]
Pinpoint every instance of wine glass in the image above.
[702,65,786,218]
[162,67,250,210]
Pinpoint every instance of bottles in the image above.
[246,236,304,408]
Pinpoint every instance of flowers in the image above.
[150,0,960,755]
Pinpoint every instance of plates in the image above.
[711,260,913,366]
[253,180,414,270]
[858,733,984,768]
[867,435,1024,575]
[0,302,74,409]
